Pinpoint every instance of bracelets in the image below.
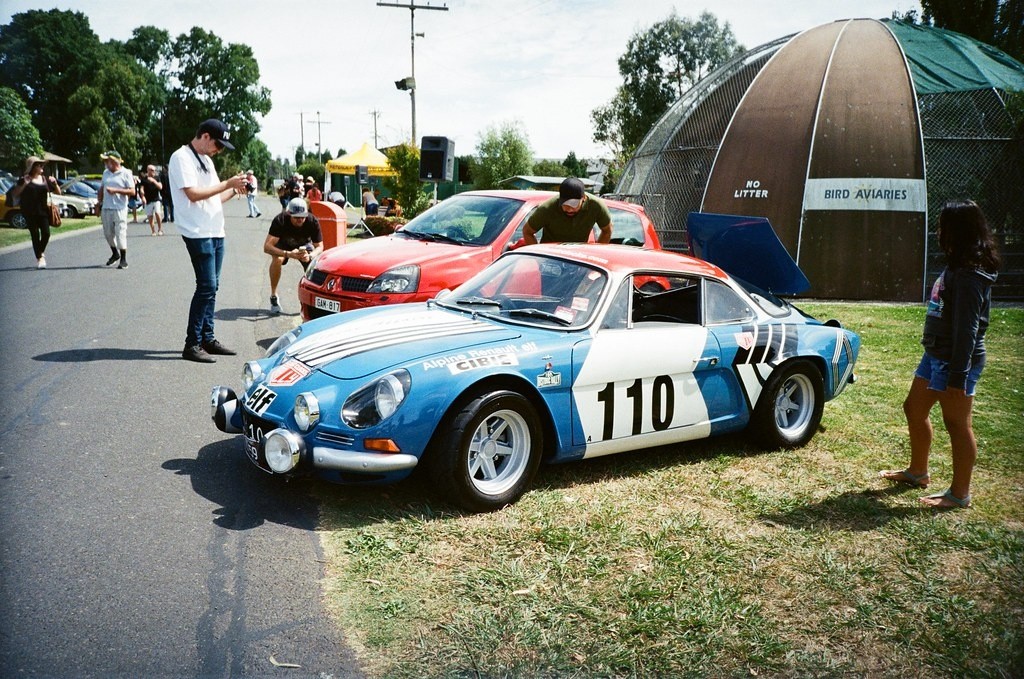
[285,251,287,257]
[233,188,237,194]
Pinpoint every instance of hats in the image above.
[292,172,315,184]
[286,198,309,218]
[559,177,584,208]
[24,155,45,175]
[204,119,235,150]
[100,149,122,163]
[246,170,253,175]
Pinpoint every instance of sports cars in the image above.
[211,242,864,512]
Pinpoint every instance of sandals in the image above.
[879,469,930,489]
[919,487,972,509]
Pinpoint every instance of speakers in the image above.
[344,176,350,186]
[355,165,368,184]
[419,136,455,183]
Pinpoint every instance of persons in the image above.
[329,190,345,209]
[523,176,613,308]
[264,198,324,314]
[245,170,262,218]
[279,172,304,208]
[141,165,162,236]
[168,118,249,362]
[11,156,61,269]
[362,187,379,215]
[94,150,135,269]
[381,196,389,206]
[159,163,175,223]
[303,175,322,201]
[129,176,141,224]
[879,199,1000,507]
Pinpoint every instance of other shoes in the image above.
[130,215,174,237]
[36,253,46,269]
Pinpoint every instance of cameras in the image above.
[246,182,256,193]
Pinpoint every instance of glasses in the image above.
[214,140,225,150]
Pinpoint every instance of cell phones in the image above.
[299,246,306,253]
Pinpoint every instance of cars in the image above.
[296,189,673,325]
[0,169,143,230]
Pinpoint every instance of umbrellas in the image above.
[325,141,399,176]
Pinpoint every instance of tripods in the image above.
[343,185,356,211]
[346,184,375,237]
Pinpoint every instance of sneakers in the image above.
[271,305,280,316]
[203,339,237,355]
[106,254,120,266]
[182,346,216,362]
[118,261,128,270]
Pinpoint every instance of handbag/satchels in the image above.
[5,176,32,207]
[47,204,61,226]
